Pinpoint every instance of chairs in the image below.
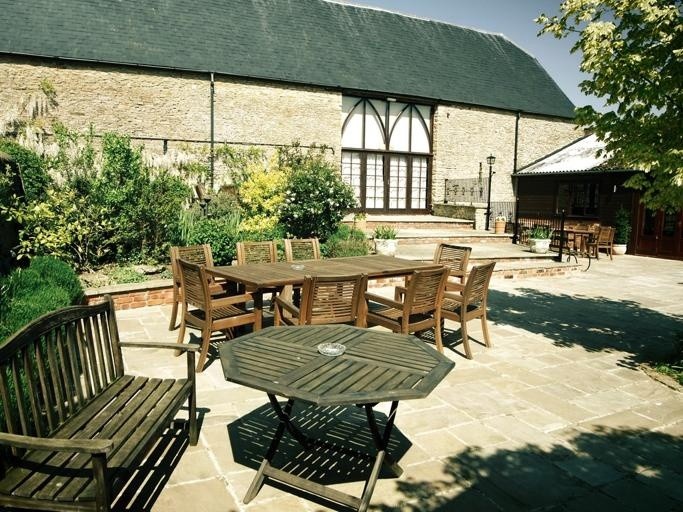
[602,228,616,256]
[271,273,367,328]
[168,244,217,329]
[412,242,471,295]
[365,265,450,353]
[436,260,496,360]
[587,227,613,260]
[174,257,263,371]
[235,241,277,263]
[284,237,320,261]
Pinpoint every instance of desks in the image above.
[555,228,592,257]
[217,323,456,511]
[207,256,443,333]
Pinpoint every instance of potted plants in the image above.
[496,217,506,233]
[610,203,632,255]
[352,210,368,232]
[526,225,555,254]
[372,224,399,257]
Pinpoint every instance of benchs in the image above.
[1,294,201,511]
